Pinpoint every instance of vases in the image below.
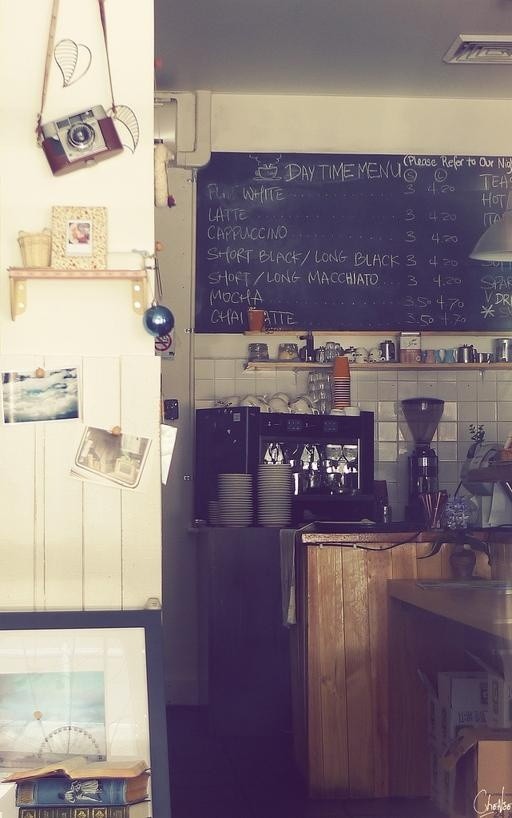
[449,549,477,577]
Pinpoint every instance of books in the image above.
[0,754,153,818]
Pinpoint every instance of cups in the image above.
[418,490,448,530]
[340,345,385,364]
[458,345,479,362]
[380,342,396,362]
[223,391,320,415]
[494,336,512,363]
[332,354,354,409]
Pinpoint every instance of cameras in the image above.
[41,105,123,178]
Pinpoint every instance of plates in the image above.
[205,462,293,530]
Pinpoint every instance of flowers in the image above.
[443,494,476,546]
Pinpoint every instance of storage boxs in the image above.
[414,665,512,817]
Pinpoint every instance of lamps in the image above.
[465,182,512,266]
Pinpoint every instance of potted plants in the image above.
[16,226,50,267]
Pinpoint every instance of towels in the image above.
[278,529,298,629]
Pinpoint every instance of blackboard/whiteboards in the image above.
[191,152,512,337]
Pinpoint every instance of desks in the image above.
[384,576,512,817]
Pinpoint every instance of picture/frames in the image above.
[50,205,109,270]
[0,606,179,818]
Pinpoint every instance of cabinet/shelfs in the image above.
[242,330,512,372]
[193,523,291,749]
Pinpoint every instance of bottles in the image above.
[247,340,298,363]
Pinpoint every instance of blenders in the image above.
[401,397,446,521]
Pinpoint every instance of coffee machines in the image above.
[196,408,376,533]
